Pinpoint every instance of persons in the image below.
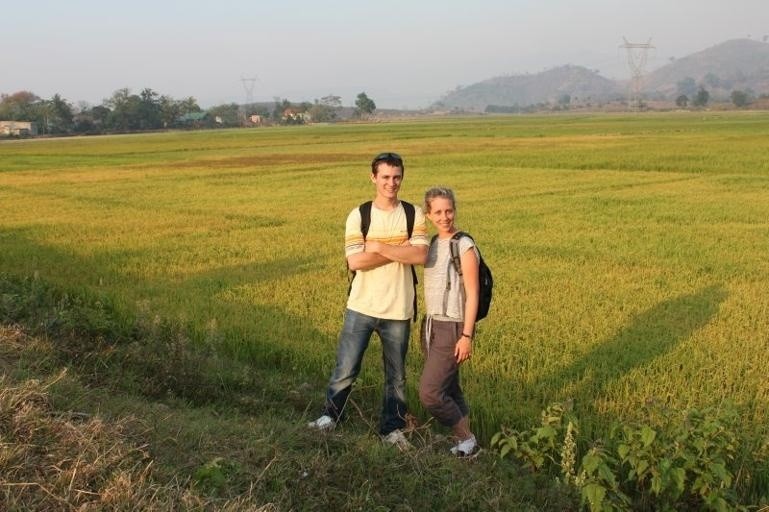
[308,154,430,454]
[419,188,480,462]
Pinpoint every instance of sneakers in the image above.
[449,435,478,457]
[308,416,337,433]
[383,429,417,452]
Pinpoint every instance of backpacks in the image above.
[428,232,494,323]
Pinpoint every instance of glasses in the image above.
[372,153,402,162]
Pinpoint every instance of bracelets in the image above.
[462,333,473,339]
